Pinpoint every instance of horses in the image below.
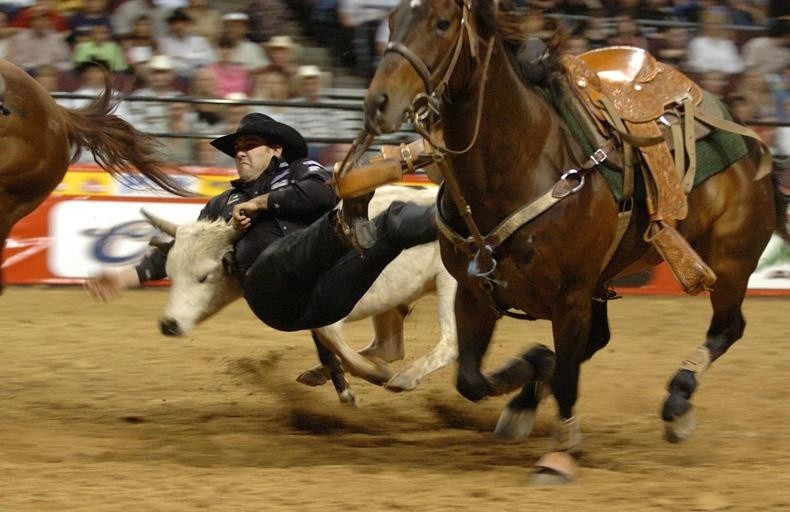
[362,0,790,478]
[0,58,210,296]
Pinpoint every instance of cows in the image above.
[139,183,459,406]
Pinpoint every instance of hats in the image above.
[75,7,332,79]
[209,114,309,163]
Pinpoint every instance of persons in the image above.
[83,113,440,334]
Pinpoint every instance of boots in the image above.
[331,190,379,247]
